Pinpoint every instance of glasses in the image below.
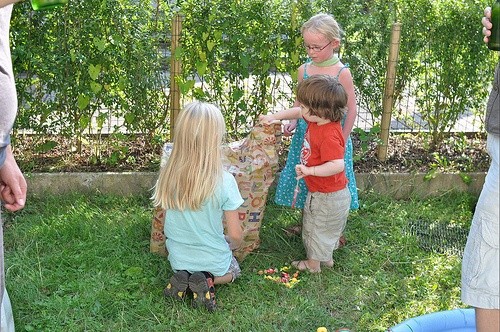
[304,41,332,52]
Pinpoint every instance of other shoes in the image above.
[291,259,322,274]
[283,223,303,236]
[320,260,334,268]
[338,234,346,248]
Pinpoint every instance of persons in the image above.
[0,0,27,332]
[145,101,245,312]
[257,12,358,274]
[388,0,499,331]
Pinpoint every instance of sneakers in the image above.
[163,270,189,301]
[189,271,217,314]
